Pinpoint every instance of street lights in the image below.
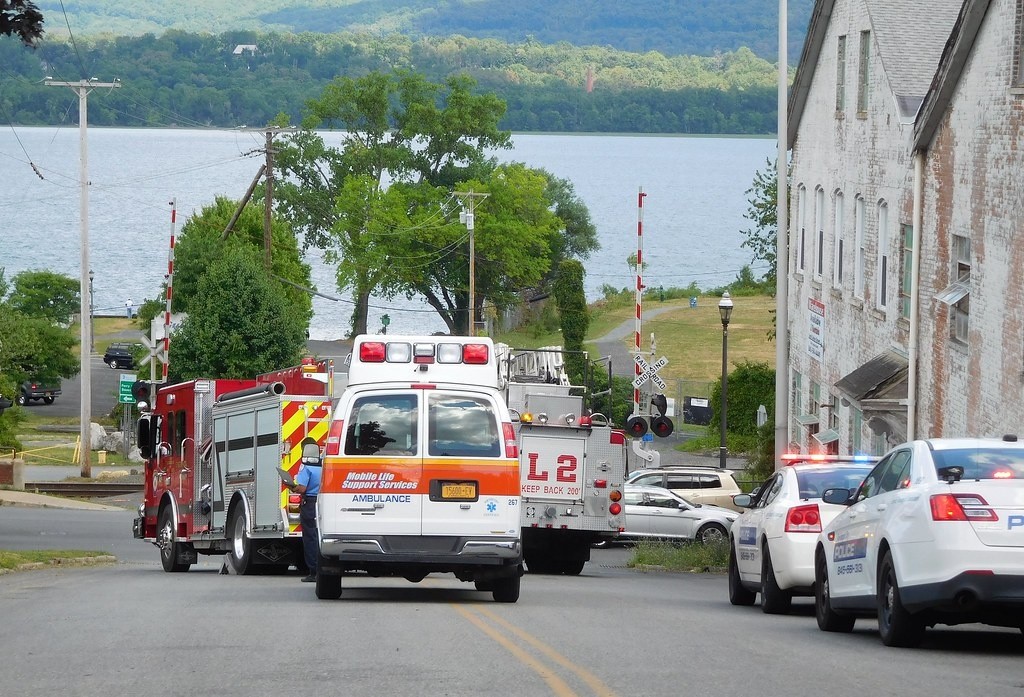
[715,289,734,469]
[88,269,95,353]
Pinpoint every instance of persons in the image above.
[284,437,322,582]
[125,298,133,318]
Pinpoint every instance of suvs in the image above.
[812,434,1023,651]
[728,453,883,616]
[102,343,136,369]
[624,464,746,515]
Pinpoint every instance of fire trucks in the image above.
[128,333,628,577]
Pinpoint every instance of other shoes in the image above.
[301,575,316,582]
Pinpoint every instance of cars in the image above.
[300,377,527,603]
[591,485,741,549]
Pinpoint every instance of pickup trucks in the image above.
[16,380,62,406]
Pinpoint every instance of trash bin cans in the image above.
[98,450,106,464]
[689,296,697,308]
